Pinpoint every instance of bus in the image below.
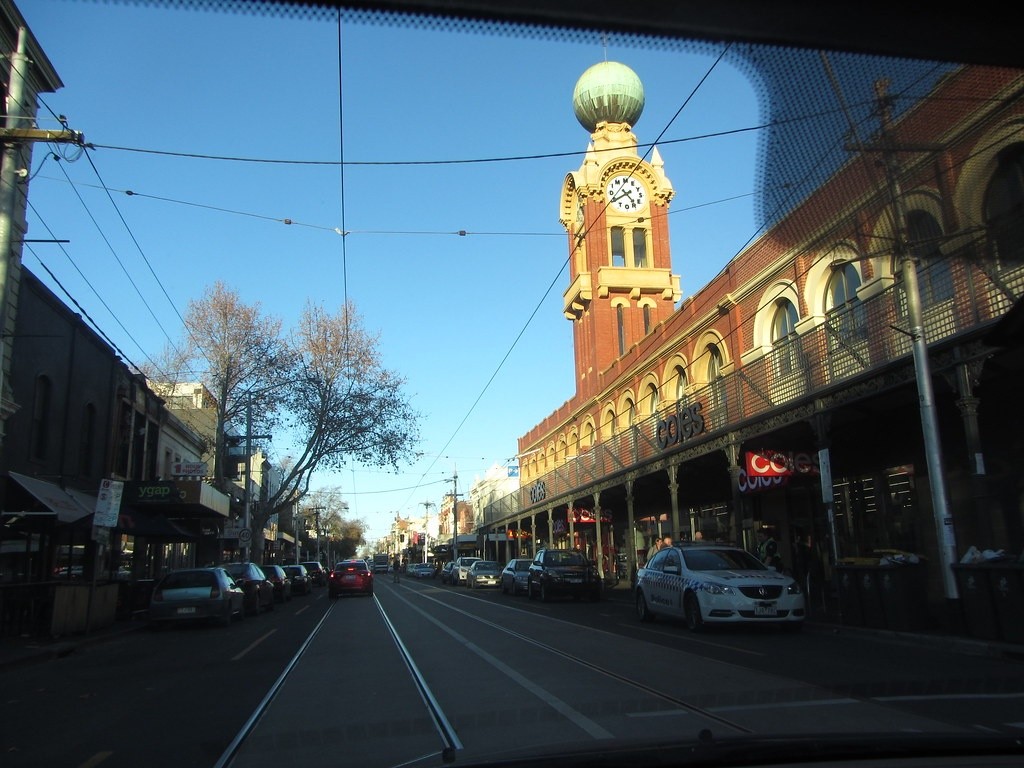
[373,554,389,574]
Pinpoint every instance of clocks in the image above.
[606,175,647,214]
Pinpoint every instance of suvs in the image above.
[451,557,484,585]
[527,548,596,600]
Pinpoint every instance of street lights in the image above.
[317,507,349,562]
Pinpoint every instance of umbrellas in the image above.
[107,504,199,579]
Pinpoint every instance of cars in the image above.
[390,561,455,584]
[216,561,331,616]
[466,561,502,588]
[148,567,244,626]
[633,542,806,631]
[499,558,533,595]
[328,556,373,597]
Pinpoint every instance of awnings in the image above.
[0,470,98,526]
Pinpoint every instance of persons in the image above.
[647,538,662,559]
[757,529,784,574]
[660,538,672,550]
[393,559,400,583]
[695,532,705,542]
